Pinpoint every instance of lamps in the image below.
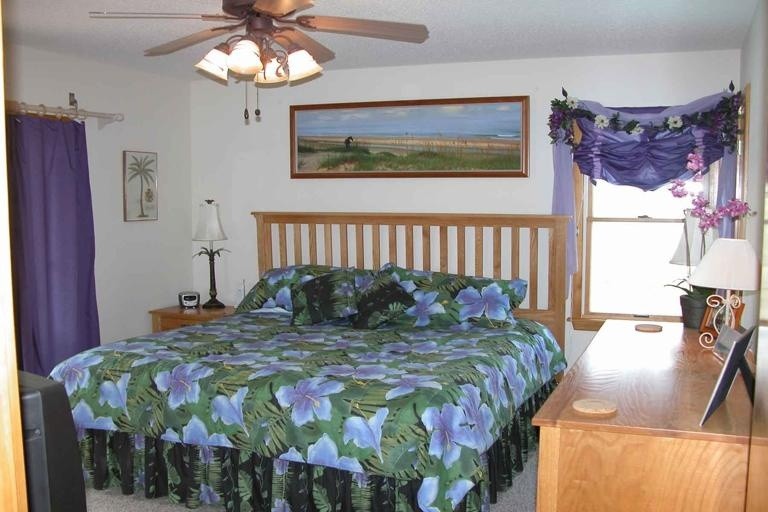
[689,236,758,351]
[193,198,228,310]
[194,17,324,85]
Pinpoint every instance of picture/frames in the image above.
[123,150,158,221]
[289,95,530,176]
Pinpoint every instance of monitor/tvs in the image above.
[18,370,87,512]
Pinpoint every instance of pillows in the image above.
[235,263,529,333]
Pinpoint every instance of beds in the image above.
[48,211,574,512]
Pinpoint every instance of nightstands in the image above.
[148,303,239,335]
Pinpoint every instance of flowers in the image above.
[663,146,754,298]
[548,80,747,155]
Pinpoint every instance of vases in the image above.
[681,295,711,329]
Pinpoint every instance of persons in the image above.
[345,135,354,152]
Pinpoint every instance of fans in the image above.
[88,0,430,85]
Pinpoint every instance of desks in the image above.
[529,318,753,512]
